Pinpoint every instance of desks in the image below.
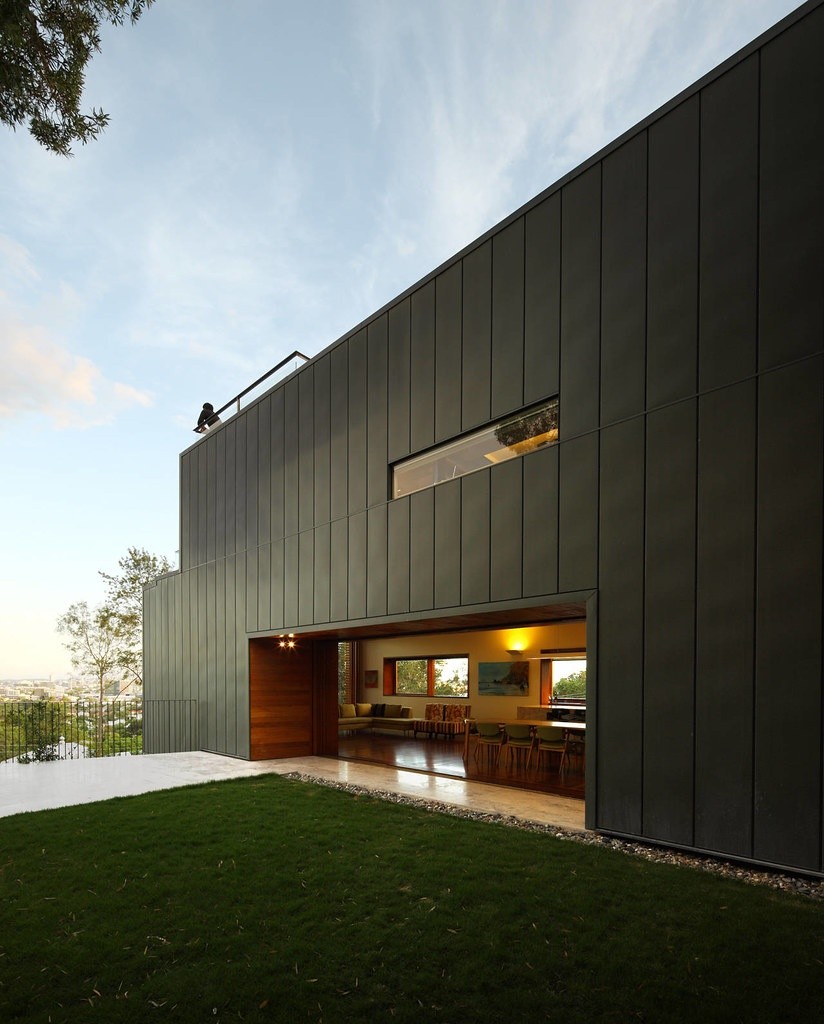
[464,718,586,762]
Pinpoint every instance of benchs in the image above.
[338,706,425,737]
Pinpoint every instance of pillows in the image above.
[339,704,356,718]
[356,703,372,717]
[371,704,386,717]
[385,704,402,718]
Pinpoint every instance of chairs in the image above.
[435,704,472,739]
[476,723,570,775]
[414,703,446,739]
